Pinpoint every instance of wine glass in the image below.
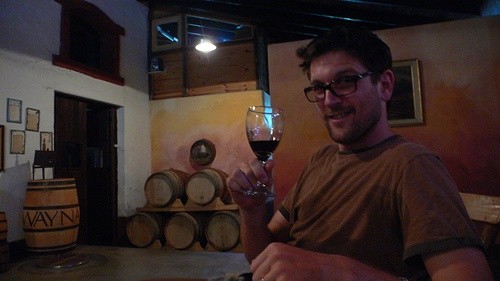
[242,105,283,196]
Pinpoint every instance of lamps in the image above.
[195,21,217,53]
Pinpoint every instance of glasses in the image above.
[302,71,374,104]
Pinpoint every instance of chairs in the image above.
[457,193,500,259]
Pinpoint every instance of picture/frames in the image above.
[6,97,23,123]
[150,12,184,54]
[0,125,5,171]
[26,107,41,132]
[10,129,27,155]
[40,130,53,152]
[386,57,425,127]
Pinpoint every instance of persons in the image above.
[226,29,494,281]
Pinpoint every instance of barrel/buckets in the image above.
[183,168,230,206]
[164,211,209,249]
[203,210,241,252]
[144,169,188,208]
[22,177,80,253]
[0,211,8,244]
[126,211,168,249]
